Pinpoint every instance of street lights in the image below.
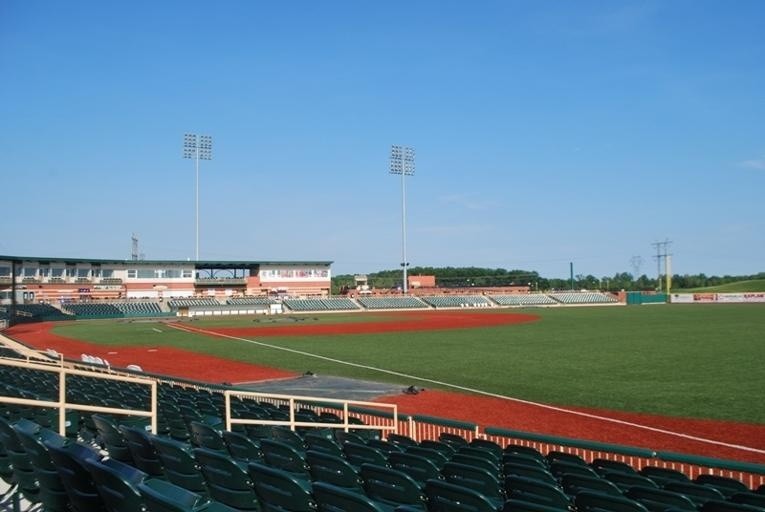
[390,144,416,293]
[182,133,213,260]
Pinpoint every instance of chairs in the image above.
[0,362,765,512]
